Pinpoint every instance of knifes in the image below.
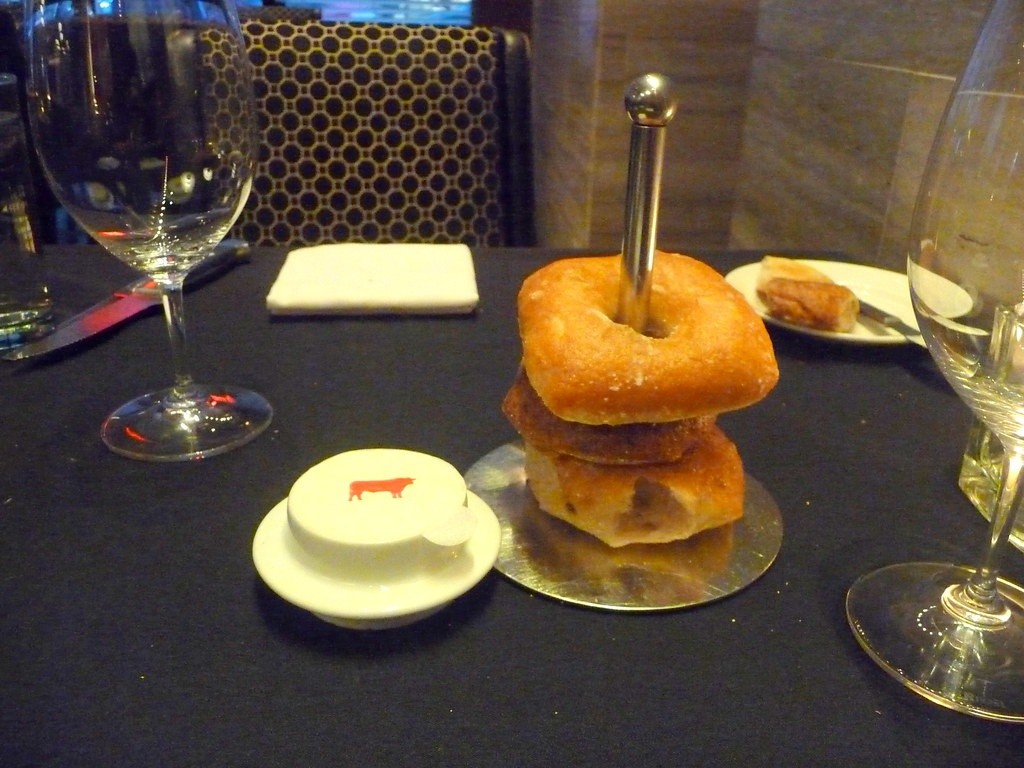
[856,299,928,347]
[0,239,253,361]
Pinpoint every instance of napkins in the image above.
[265,244,480,316]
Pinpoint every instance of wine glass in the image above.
[845,0,1024,722]
[26,1,273,463]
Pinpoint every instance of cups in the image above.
[957,304,1024,553]
[0,185,55,351]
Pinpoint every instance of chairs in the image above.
[206,7,531,249]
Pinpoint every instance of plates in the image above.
[251,488,502,630]
[724,258,973,343]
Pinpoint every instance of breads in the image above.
[757,255,860,333]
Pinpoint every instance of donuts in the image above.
[500,248,781,549]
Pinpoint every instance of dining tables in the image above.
[0,236,1024,768]
